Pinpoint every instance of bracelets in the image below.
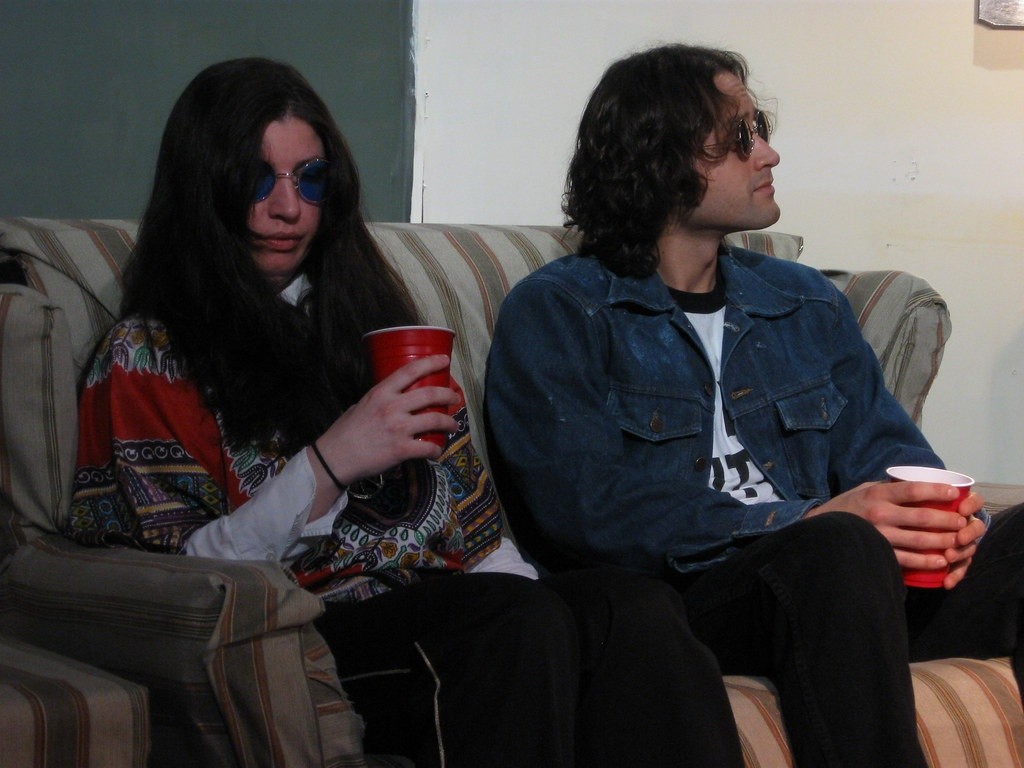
[311,440,349,492]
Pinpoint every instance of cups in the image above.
[887,466,976,589]
[362,327,457,449]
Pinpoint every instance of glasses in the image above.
[251,157,330,205]
[705,111,771,161]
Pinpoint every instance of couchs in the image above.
[0,218,1024,768]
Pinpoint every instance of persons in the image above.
[483,43,1024,768]
[68,56,746,768]
